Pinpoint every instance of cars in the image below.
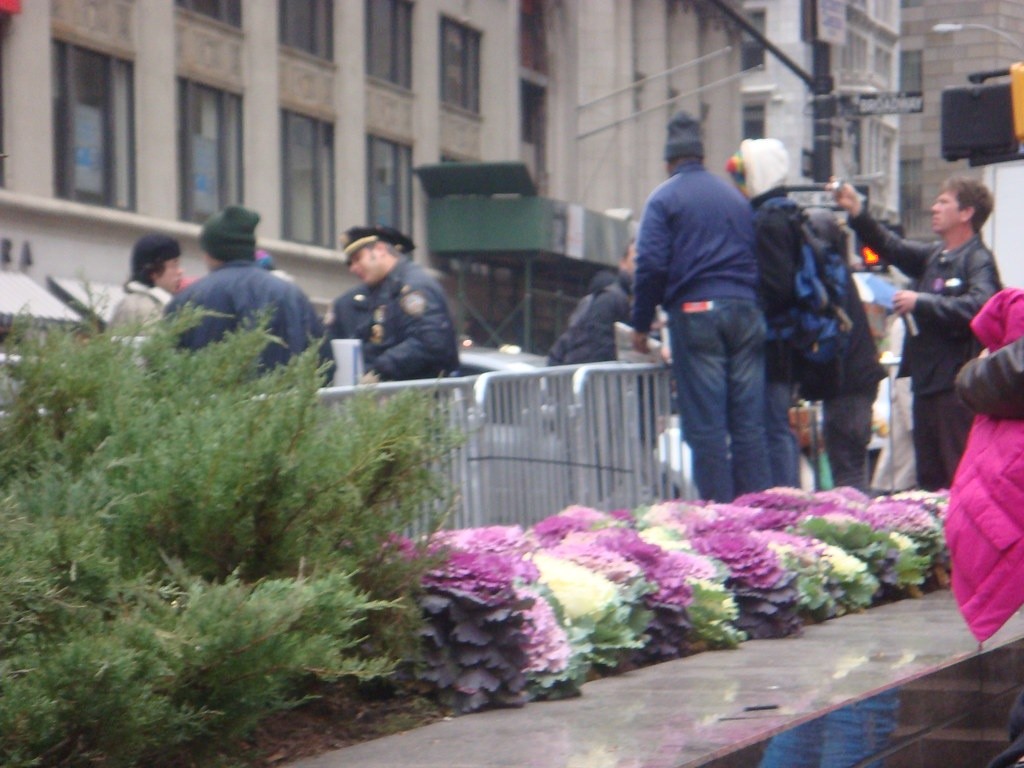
[456,345,572,521]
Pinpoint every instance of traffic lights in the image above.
[859,245,885,267]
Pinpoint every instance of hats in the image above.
[380,226,415,255]
[197,204,260,262]
[255,250,274,271]
[337,225,386,262]
[662,110,704,160]
[724,151,748,194]
[590,271,614,296]
[130,233,179,276]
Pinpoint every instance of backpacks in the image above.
[753,198,850,360]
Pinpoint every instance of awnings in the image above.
[0,271,82,323]
[51,277,128,324]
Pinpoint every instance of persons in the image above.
[808,208,887,489]
[870,309,920,492]
[728,138,811,489]
[632,131,774,502]
[760,687,899,768]
[827,175,1002,491]
[324,223,461,382]
[106,235,182,337]
[162,207,337,388]
[548,235,637,366]
[954,335,1024,418]
